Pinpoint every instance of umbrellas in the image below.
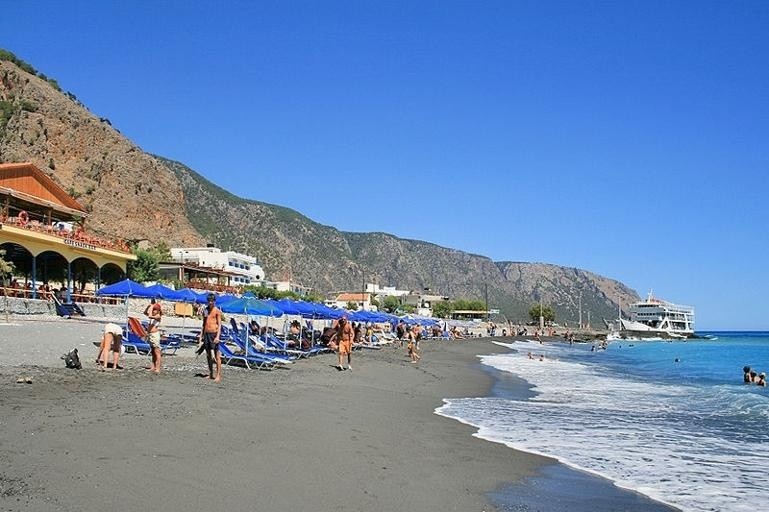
[449,319,466,328]
[466,321,480,328]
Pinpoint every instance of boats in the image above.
[602,288,695,340]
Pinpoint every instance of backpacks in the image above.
[60,349,81,368]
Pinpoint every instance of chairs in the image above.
[102,318,477,372]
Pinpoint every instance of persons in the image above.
[756,372,766,386]
[527,352,534,359]
[539,355,544,361]
[742,365,751,382]
[143,301,163,374]
[328,315,356,372]
[487,323,608,353]
[750,370,758,384]
[448,326,474,341]
[201,294,223,384]
[98,319,124,371]
[407,329,421,364]
